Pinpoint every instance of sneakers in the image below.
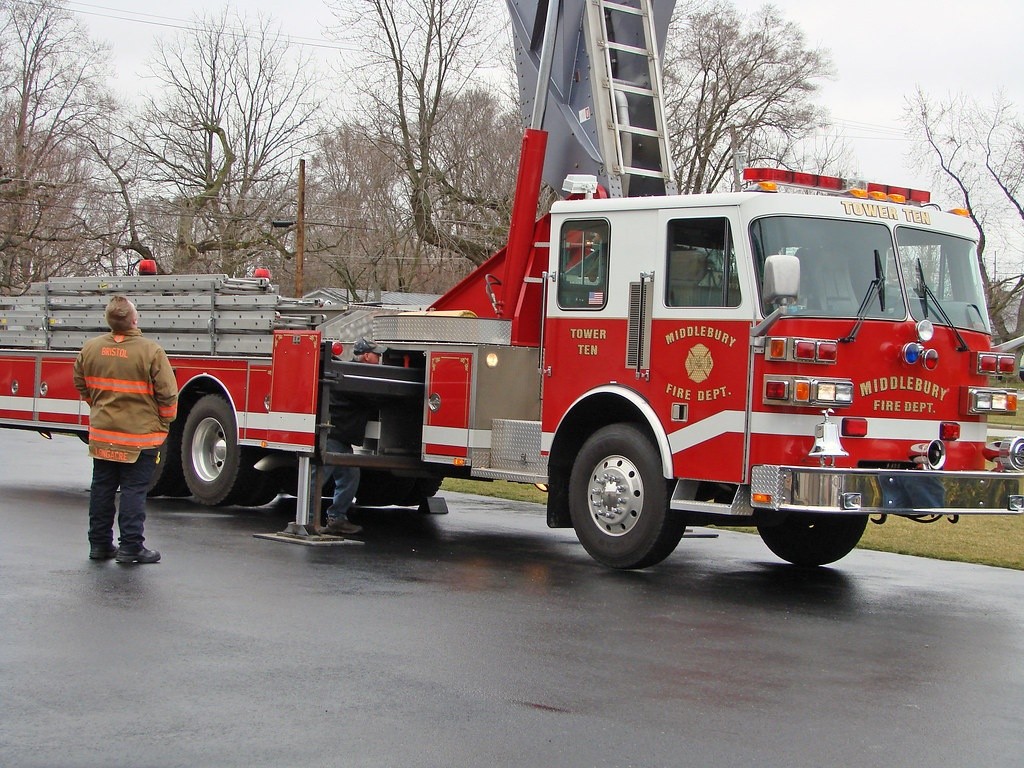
[327,515,363,534]
[312,523,327,533]
[116,545,162,564]
[89,541,120,559]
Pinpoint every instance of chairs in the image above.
[666,250,710,306]
[804,244,861,316]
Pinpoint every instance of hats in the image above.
[354,338,388,353]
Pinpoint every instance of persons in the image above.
[306,338,388,533]
[73,296,179,563]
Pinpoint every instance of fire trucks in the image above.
[0,1,1023,574]
